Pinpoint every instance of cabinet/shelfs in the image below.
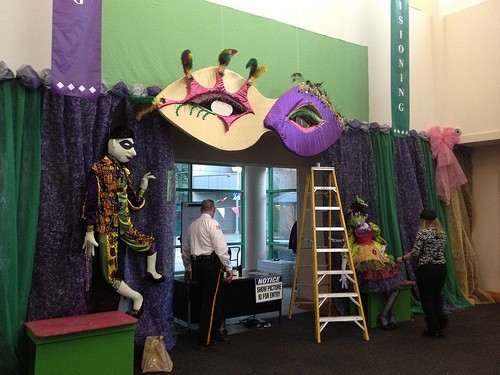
[26,324,136,375]
[349,283,414,327]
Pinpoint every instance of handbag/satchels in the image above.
[141,335,173,373]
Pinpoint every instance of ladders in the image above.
[288,167,369,343]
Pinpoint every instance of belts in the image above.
[191,256,211,260]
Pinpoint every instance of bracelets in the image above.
[402,254,406,261]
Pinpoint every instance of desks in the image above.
[172,268,283,335]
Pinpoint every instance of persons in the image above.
[181,199,233,350]
[340,197,406,330]
[397,209,448,339]
[81,125,166,319]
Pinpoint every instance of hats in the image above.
[419,208,437,220]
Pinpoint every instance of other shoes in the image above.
[422,322,449,339]
